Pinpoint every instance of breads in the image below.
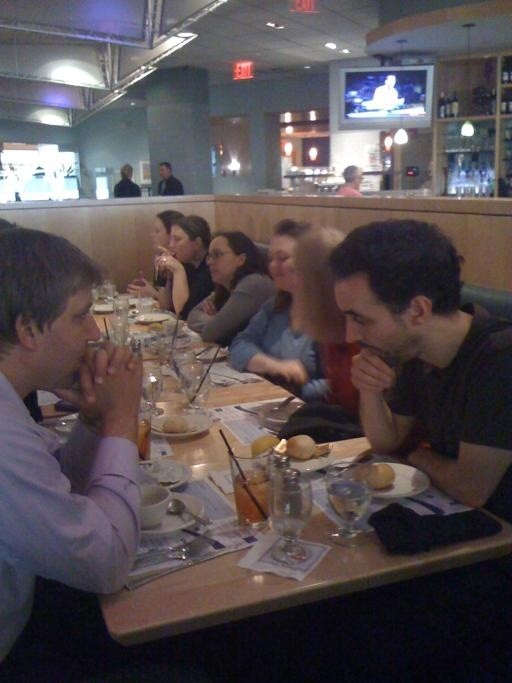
[250,437,278,460]
[369,465,395,489]
[161,415,188,433]
[148,323,162,334]
[287,435,315,460]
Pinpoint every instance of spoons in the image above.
[168,498,214,529]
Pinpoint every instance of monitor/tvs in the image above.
[328,56,439,131]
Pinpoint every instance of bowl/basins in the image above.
[136,485,170,529]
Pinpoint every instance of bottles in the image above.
[438,89,460,119]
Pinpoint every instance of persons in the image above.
[229,219,329,398]
[328,219,512,509]
[187,229,274,346]
[373,74,405,107]
[127,210,184,299]
[291,225,361,411]
[0,218,44,424]
[157,162,183,195]
[0,226,141,683]
[153,215,215,319]
[335,164,366,197]
[113,163,142,197]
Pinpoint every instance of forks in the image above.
[132,529,216,569]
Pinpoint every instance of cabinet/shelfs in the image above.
[392,49,512,197]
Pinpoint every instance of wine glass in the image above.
[182,361,210,415]
[142,368,170,414]
[97,279,191,356]
[323,463,370,547]
[270,478,311,562]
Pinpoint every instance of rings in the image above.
[162,256,167,261]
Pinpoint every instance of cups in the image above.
[138,414,154,462]
[230,449,272,528]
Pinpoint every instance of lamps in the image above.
[373,23,476,151]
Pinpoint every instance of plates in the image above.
[137,491,204,537]
[358,462,430,497]
[151,412,213,439]
[136,458,191,490]
[258,401,305,423]
